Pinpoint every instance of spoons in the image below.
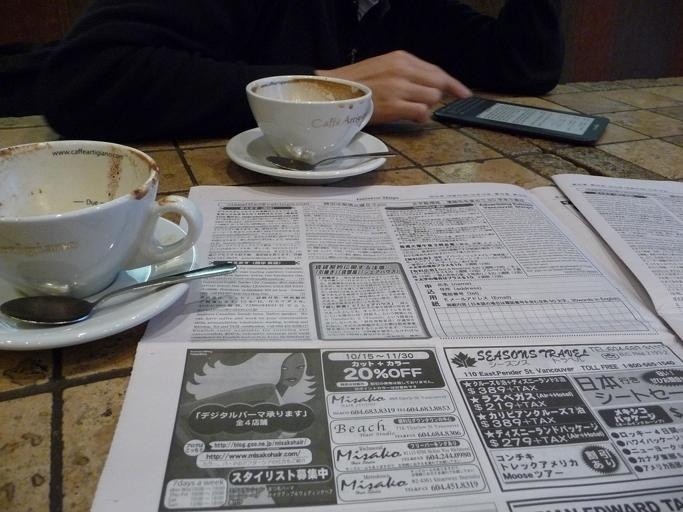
[264,152,402,171]
[0,259,236,325]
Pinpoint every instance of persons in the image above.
[33,1,566,147]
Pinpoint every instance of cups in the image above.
[247,73,374,165]
[0,138,202,296]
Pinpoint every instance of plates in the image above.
[0,218,198,352]
[226,126,390,182]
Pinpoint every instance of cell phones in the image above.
[432,96,610,146]
